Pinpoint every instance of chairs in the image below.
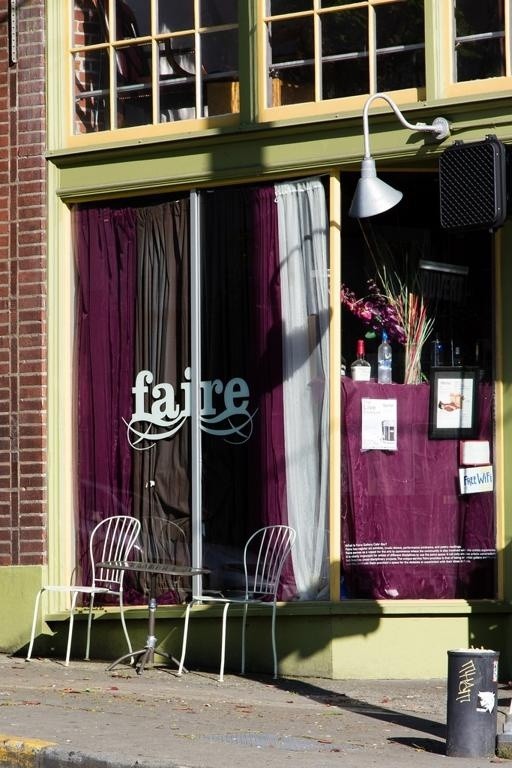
[27,514,142,669]
[96,0,232,126]
[122,510,226,605]
[173,525,296,687]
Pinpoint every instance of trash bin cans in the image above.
[446,649,500,759]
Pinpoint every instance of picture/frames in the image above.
[427,368,474,440]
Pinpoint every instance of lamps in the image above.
[346,93,449,219]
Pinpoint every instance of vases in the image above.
[400,340,421,386]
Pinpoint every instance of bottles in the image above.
[454,344,462,365]
[352,338,371,381]
[431,336,444,366]
[376,332,393,384]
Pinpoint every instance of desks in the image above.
[94,559,211,676]
[206,79,315,116]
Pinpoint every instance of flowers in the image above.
[336,257,438,360]
